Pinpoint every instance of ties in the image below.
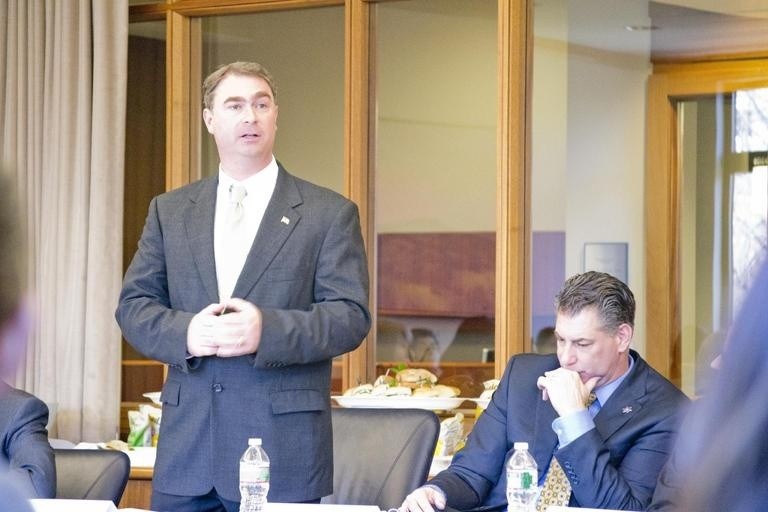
[536,392,598,512]
[221,183,246,308]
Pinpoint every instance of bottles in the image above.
[237,436,270,511]
[504,441,539,511]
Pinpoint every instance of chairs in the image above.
[55,450,130,508]
[321,408,440,512]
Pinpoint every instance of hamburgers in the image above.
[344,365,462,399]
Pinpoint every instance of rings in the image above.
[238,335,246,347]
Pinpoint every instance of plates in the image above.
[331,396,465,409]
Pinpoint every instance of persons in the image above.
[397,271,693,512]
[0,378,57,510]
[115,61,370,512]
